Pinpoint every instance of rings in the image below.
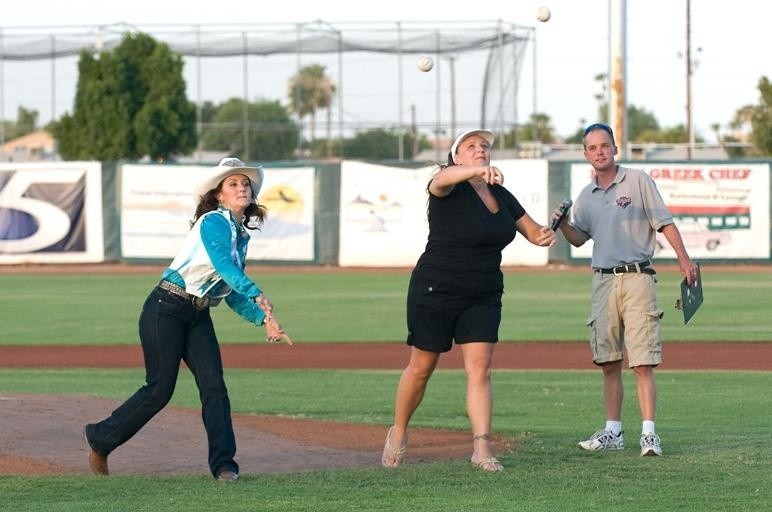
[694,273,697,275]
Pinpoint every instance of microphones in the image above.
[551,197,572,231]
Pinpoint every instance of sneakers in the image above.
[639,431,663,457]
[577,428,624,451]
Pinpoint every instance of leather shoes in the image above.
[217,470,240,483]
[80,423,111,477]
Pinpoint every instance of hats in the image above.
[450,127,495,166]
[192,157,266,208]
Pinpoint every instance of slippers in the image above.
[379,423,407,469]
[470,452,505,473]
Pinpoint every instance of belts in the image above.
[158,279,225,312]
[593,260,656,275]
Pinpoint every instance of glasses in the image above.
[583,123,611,135]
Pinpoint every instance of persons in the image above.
[551,124,699,458]
[381,129,558,474]
[82,157,293,483]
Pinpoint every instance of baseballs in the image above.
[535,7,551,22]
[416,56,433,72]
